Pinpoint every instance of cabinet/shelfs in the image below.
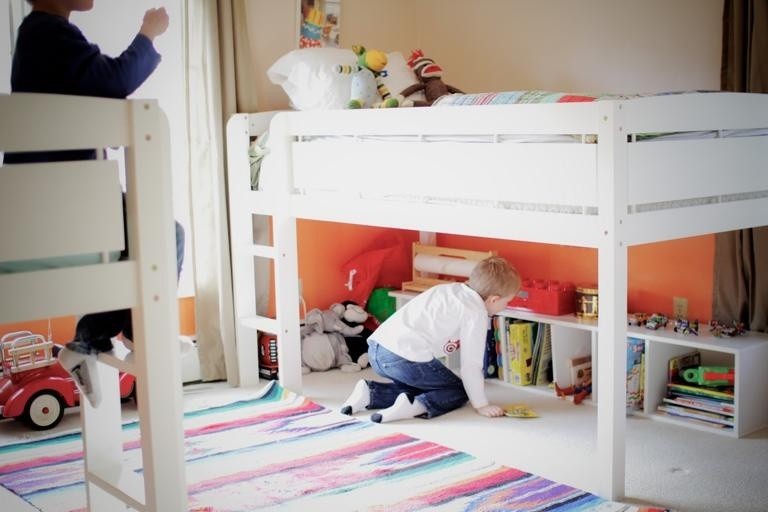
[387,292,766,440]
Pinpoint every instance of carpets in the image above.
[0,381,690,512]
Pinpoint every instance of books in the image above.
[481,316,552,391]
[654,360,734,429]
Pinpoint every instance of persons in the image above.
[15,1,182,410]
[337,254,525,424]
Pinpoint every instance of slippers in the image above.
[64,333,114,354]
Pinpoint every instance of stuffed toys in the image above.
[333,43,398,111]
[296,303,359,373]
[555,376,593,404]
[394,50,465,108]
[335,299,378,368]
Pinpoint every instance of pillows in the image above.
[266,47,426,112]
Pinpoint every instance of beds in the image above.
[219,90,766,503]
[0,94,193,512]
